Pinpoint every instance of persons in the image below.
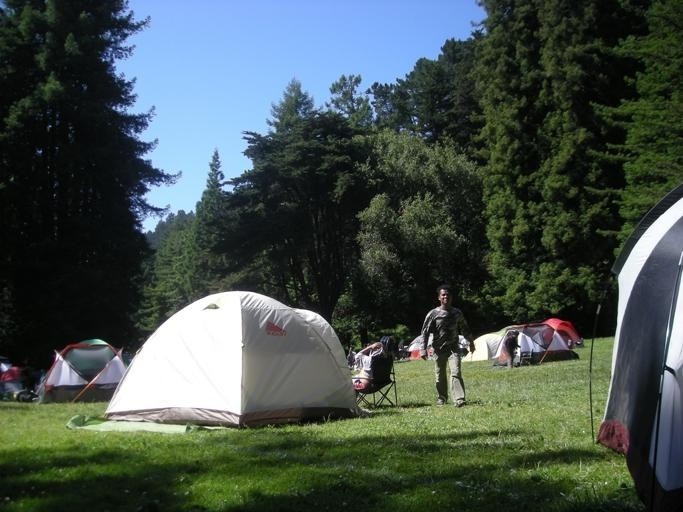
[350,335,395,398]
[419,286,475,407]
[347,348,354,367]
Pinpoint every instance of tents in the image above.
[34,338,127,403]
[0,356,24,402]
[103,290,372,429]
[406,317,585,370]
[588,182,683,510]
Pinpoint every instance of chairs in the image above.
[520,348,533,366]
[355,355,398,410]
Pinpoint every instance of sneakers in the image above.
[437,401,444,406]
[455,400,466,407]
